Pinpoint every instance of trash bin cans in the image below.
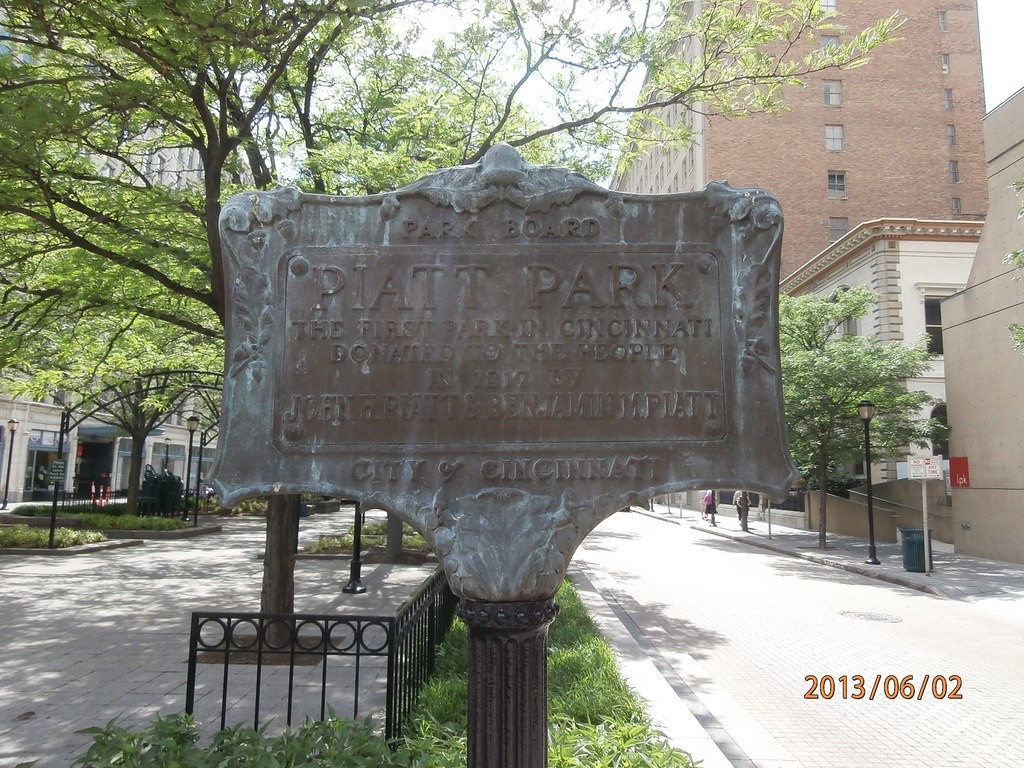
[899,527,933,572]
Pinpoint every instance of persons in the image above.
[733,490,751,514]
[699,489,717,521]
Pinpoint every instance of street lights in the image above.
[181,415,200,523]
[164,436,173,470]
[0,418,21,511]
[855,398,881,566]
